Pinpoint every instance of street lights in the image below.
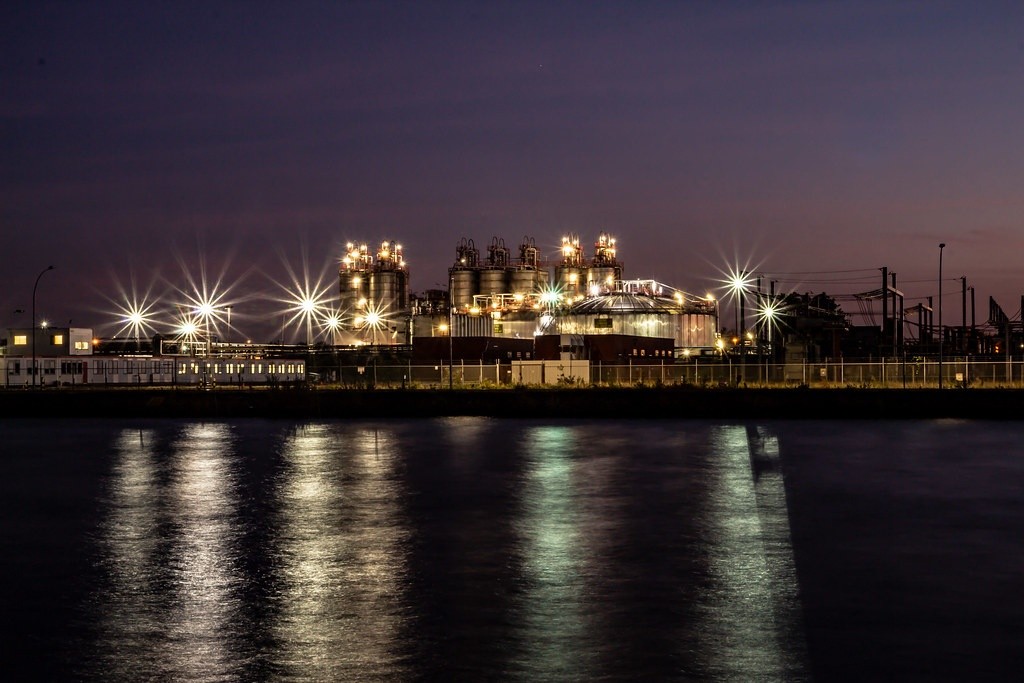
[32,261,63,388]
[201,302,213,358]
[368,311,380,387]
[938,240,946,392]
